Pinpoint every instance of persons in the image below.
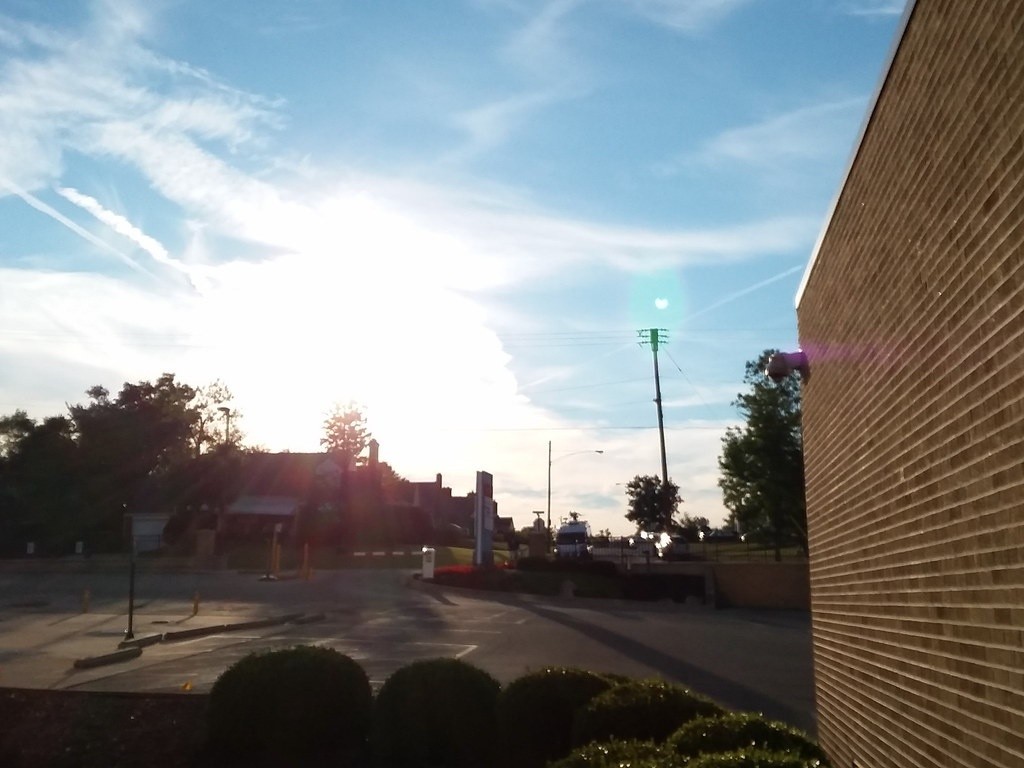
[508,534,519,560]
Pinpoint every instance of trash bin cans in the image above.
[195,529,216,555]
